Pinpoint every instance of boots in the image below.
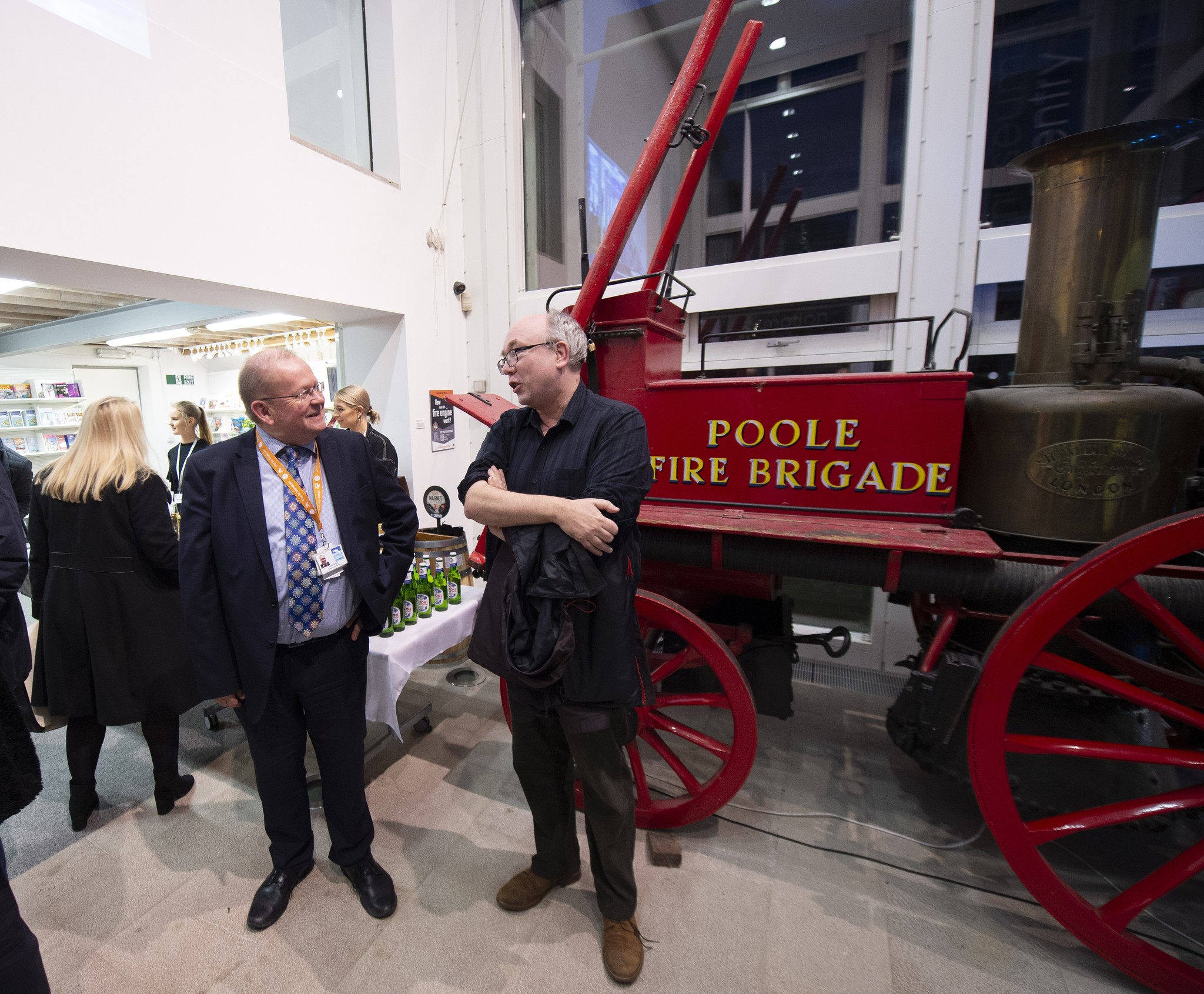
[153,763,195,815]
[69,779,98,832]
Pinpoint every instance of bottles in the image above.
[422,554,434,608]
[433,557,448,612]
[446,552,461,605]
[417,562,432,618]
[379,553,419,638]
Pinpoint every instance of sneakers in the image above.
[497,865,581,911]
[602,917,661,982]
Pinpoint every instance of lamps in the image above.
[105,313,330,362]
[97,348,128,359]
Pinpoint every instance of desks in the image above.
[302,584,485,788]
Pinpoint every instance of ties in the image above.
[278,446,325,637]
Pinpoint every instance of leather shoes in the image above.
[247,858,314,929]
[340,854,398,919]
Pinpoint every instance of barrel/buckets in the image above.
[414,527,474,670]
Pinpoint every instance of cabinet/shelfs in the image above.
[196,407,256,444]
[0,368,90,460]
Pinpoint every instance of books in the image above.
[0,410,83,453]
[0,382,85,399]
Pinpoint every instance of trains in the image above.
[420,2,1203,994]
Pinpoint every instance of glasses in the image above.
[498,341,556,375]
[259,382,324,402]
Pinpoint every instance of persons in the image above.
[0,438,54,994]
[333,384,399,483]
[28,396,195,833]
[176,346,419,931]
[458,310,654,986]
[165,401,214,516]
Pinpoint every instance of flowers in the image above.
[241,417,256,430]
